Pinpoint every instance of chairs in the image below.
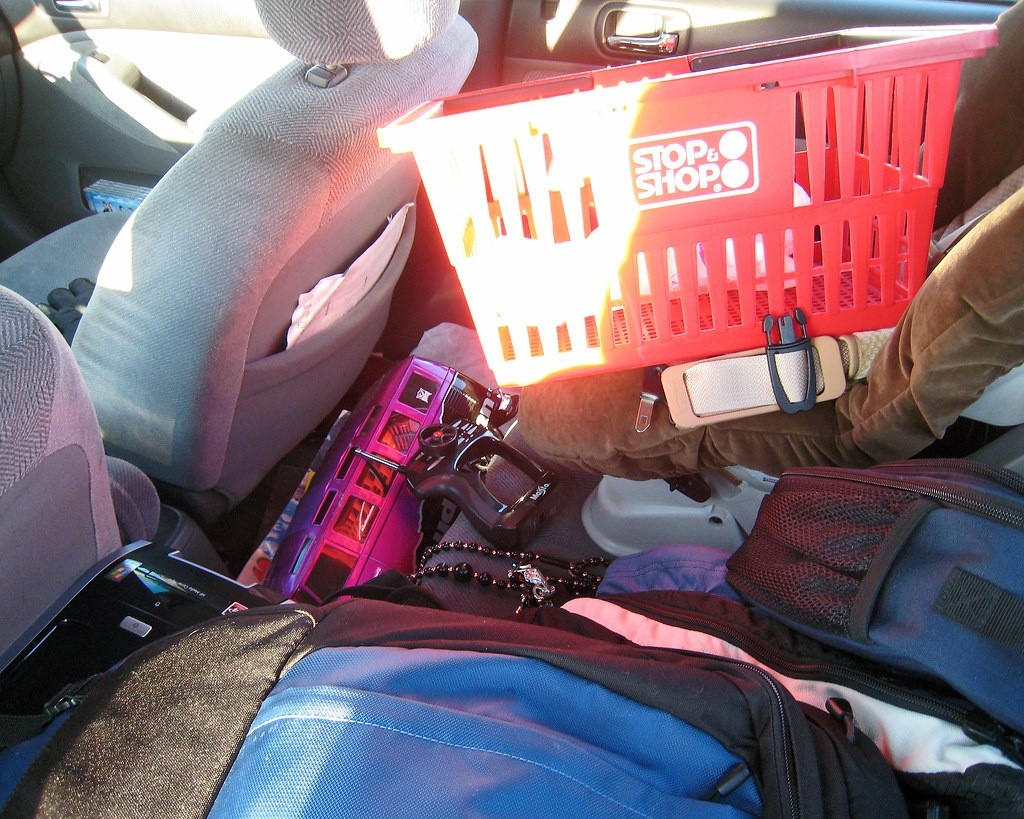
[0,0,479,521]
[0,283,163,749]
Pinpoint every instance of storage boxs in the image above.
[377,20,998,390]
